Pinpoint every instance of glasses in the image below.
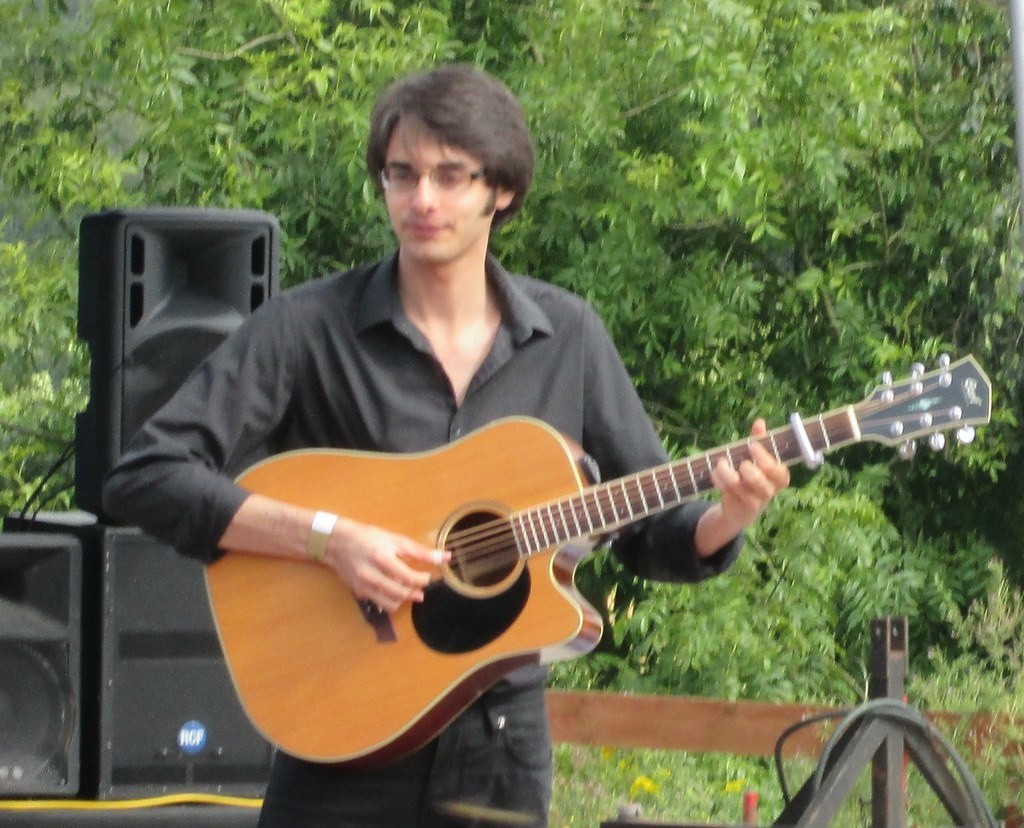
[380,163,486,192]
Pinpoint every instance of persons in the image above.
[103,66,791,828]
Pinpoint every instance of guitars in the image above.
[202,349,994,782]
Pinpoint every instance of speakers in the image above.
[0,531,81,799]
[5,510,278,805]
[74,209,283,525]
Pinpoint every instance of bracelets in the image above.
[307,511,336,562]
